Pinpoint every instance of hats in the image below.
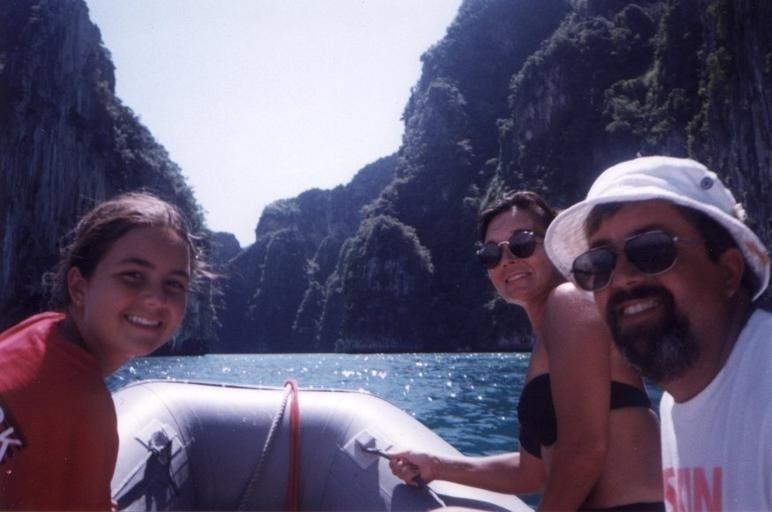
[541,152,771,303]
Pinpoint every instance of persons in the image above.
[543,154,771,512]
[0,186,243,511]
[386,190,663,512]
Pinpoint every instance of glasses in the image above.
[571,228,713,297]
[471,227,539,268]
